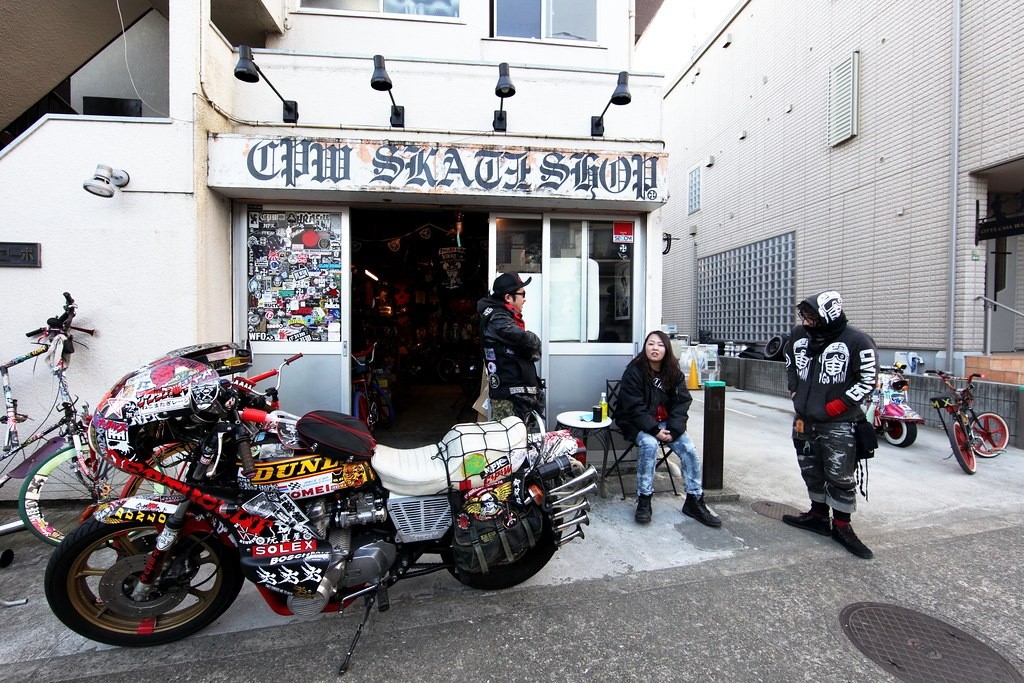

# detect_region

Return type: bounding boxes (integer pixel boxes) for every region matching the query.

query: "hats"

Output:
[493,271,532,296]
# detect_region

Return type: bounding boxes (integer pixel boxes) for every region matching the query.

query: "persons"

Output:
[615,330,723,527]
[782,290,880,558]
[477,272,541,431]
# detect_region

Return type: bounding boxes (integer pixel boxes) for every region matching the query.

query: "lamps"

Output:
[234,45,299,123]
[492,63,516,132]
[591,72,632,136]
[83,164,129,198]
[370,54,404,128]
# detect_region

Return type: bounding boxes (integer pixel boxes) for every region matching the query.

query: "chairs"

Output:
[599,379,681,501]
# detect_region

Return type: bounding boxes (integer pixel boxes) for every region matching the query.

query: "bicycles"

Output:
[925,369,1010,474]
[0,292,168,546]
[350,343,396,439]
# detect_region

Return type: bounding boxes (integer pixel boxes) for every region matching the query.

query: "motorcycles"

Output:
[41,343,599,676]
[861,363,924,448]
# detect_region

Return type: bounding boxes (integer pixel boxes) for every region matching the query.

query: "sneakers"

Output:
[783,512,831,535]
[832,519,873,558]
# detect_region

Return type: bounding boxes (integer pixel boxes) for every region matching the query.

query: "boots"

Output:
[634,489,653,522]
[683,492,720,526]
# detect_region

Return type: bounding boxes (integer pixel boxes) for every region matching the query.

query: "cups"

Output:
[592,405,602,422]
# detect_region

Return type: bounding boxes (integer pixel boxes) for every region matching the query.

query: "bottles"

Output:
[598,392,607,421]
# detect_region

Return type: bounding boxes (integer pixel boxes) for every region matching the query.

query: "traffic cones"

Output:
[686,360,702,390]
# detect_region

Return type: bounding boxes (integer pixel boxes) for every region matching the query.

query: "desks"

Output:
[552,411,612,498]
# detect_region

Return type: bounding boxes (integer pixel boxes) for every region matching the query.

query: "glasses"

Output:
[505,290,525,298]
[798,310,819,325]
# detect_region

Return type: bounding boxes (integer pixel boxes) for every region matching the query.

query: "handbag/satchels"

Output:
[296,410,376,463]
[513,388,545,423]
[853,419,878,459]
[450,497,542,573]
